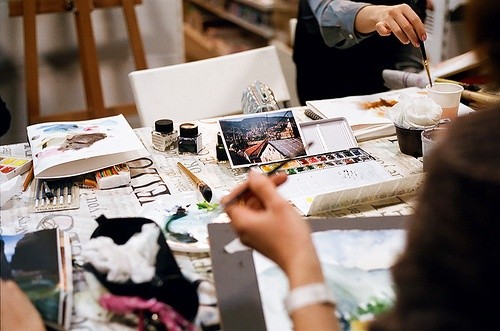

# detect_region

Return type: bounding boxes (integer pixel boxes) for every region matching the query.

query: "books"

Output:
[304,96,396,142]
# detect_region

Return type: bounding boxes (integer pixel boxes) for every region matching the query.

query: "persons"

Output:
[222,1,499,331]
[293,0,427,105]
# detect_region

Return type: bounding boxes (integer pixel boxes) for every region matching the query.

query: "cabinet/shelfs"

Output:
[183,0,298,64]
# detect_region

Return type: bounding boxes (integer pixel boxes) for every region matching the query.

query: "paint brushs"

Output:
[21,165,34,191]
[436,78,481,92]
[177,161,213,204]
[35,177,73,206]
[305,109,325,120]
[98,163,129,179]
[415,26,433,87]
[224,142,313,209]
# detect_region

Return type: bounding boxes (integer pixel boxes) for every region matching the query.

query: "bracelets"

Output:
[284,283,335,313]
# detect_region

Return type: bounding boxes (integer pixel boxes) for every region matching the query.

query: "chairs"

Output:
[128,46,290,128]
[9,0,147,125]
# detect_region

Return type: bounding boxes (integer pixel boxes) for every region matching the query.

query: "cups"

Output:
[426,82,464,120]
[394,120,439,158]
[420,128,445,172]
